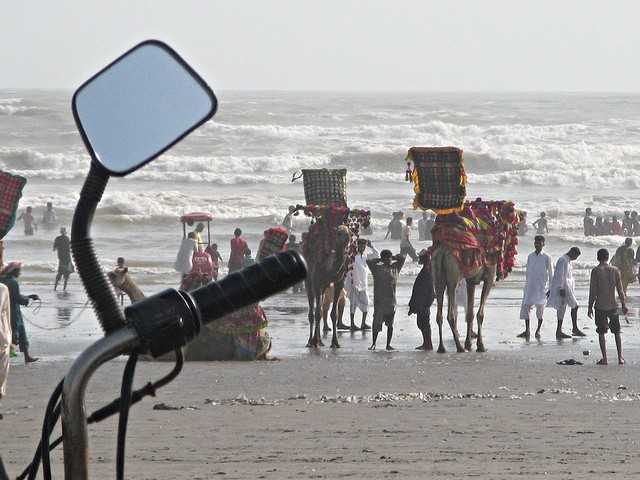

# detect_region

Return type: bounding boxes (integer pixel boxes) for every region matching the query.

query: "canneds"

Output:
[583,350,591,355]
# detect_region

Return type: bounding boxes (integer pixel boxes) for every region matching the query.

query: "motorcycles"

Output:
[0,37,308,480]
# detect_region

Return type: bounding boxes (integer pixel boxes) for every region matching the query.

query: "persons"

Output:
[52,226,75,291]
[0,283,13,420]
[194,222,205,252]
[583,207,640,236]
[281,205,296,235]
[227,228,249,275]
[407,248,436,350]
[587,249,628,364]
[365,250,406,351]
[616,238,634,297]
[384,212,403,239]
[516,235,555,339]
[17,206,38,235]
[455,278,477,338]
[348,238,380,331]
[212,243,223,272]
[173,232,198,274]
[532,211,548,234]
[517,214,528,236]
[114,256,126,306]
[255,230,307,293]
[544,246,586,338]
[322,280,350,331]
[240,249,254,269]
[0,261,40,364]
[418,212,429,241]
[400,217,418,262]
[42,201,57,224]
[424,214,436,240]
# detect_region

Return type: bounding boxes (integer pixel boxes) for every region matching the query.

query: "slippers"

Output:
[572,330,587,336]
[556,333,572,338]
[618,358,626,364]
[556,359,569,365]
[597,359,608,365]
[568,359,583,365]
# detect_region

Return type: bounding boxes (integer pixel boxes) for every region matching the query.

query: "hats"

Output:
[417,248,430,257]
